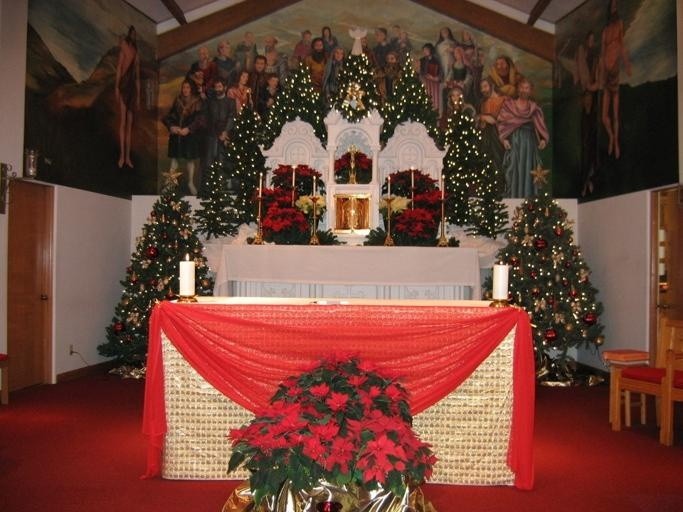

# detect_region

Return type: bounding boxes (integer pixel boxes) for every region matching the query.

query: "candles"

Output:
[177,252,196,298]
[491,262,509,301]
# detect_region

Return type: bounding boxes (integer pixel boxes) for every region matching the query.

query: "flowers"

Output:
[252,164,338,245]
[365,169,459,246]
[224,356,438,507]
[334,151,371,184]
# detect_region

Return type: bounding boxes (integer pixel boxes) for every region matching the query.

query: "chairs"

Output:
[610,314,682,446]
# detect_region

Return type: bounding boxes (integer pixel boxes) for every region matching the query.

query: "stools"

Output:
[603,349,649,428]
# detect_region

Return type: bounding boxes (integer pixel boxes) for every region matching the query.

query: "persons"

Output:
[573,42,604,196]
[114,25,141,171]
[599,1,634,160]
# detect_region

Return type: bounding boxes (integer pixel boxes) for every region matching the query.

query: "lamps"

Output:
[0,147,38,214]
